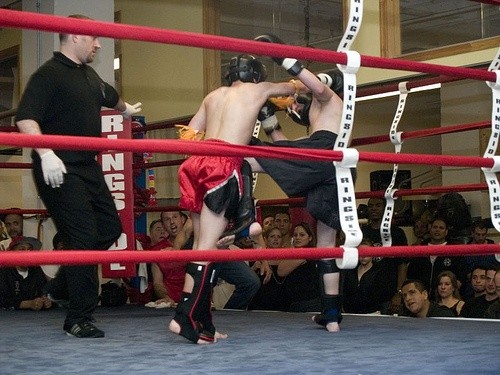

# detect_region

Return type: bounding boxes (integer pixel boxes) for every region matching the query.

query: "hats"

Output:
[7,236,42,251]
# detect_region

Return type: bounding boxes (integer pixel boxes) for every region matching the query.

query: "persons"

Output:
[169,55,344,345]
[15,15,142,337]
[217,34,356,333]
[0,194,500,320]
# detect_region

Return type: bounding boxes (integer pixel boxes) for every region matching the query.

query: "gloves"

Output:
[316,69,344,94]
[121,102,142,118]
[40,151,67,188]
[257,99,281,136]
[253,34,304,76]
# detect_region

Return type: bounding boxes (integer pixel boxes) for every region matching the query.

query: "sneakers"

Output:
[47,293,72,304]
[62,318,105,338]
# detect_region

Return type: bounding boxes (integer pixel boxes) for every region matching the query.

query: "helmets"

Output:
[284,93,314,127]
[225,54,267,86]
